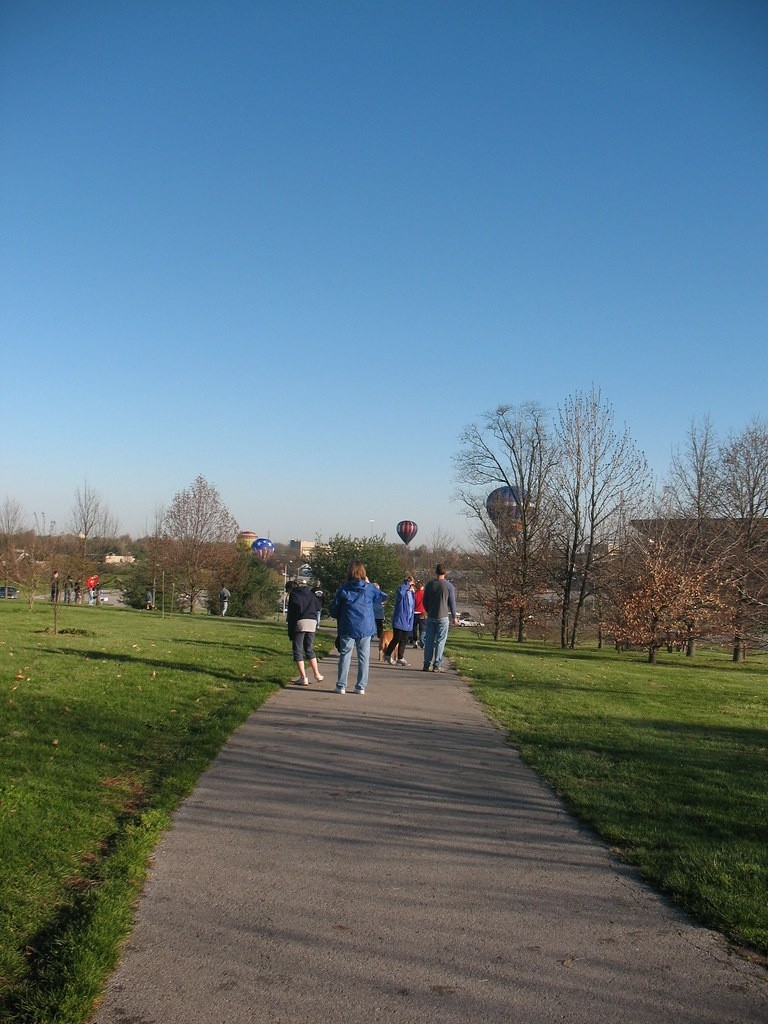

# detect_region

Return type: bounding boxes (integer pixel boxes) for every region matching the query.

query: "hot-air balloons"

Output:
[396,520,418,548]
[486,486,536,556]
[236,531,258,552]
[252,538,275,562]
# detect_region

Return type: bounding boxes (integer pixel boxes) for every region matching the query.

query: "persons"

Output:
[146,588,157,610]
[371,582,390,642]
[410,580,428,649]
[383,575,416,666]
[63,573,104,606]
[329,561,381,696]
[285,581,324,686]
[50,570,60,602]
[423,564,459,673]
[218,584,231,616]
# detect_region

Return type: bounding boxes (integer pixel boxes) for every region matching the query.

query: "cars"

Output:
[458,618,486,627]
[0,586,20,599]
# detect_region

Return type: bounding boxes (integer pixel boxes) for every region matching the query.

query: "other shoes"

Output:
[423,667,446,673]
[335,687,346,694]
[292,677,308,686]
[397,657,412,667]
[383,654,396,665]
[422,646,425,650]
[221,612,224,617]
[314,673,324,682]
[354,688,365,694]
[413,644,418,649]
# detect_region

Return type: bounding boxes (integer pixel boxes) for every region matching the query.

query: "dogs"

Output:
[377,629,397,662]
